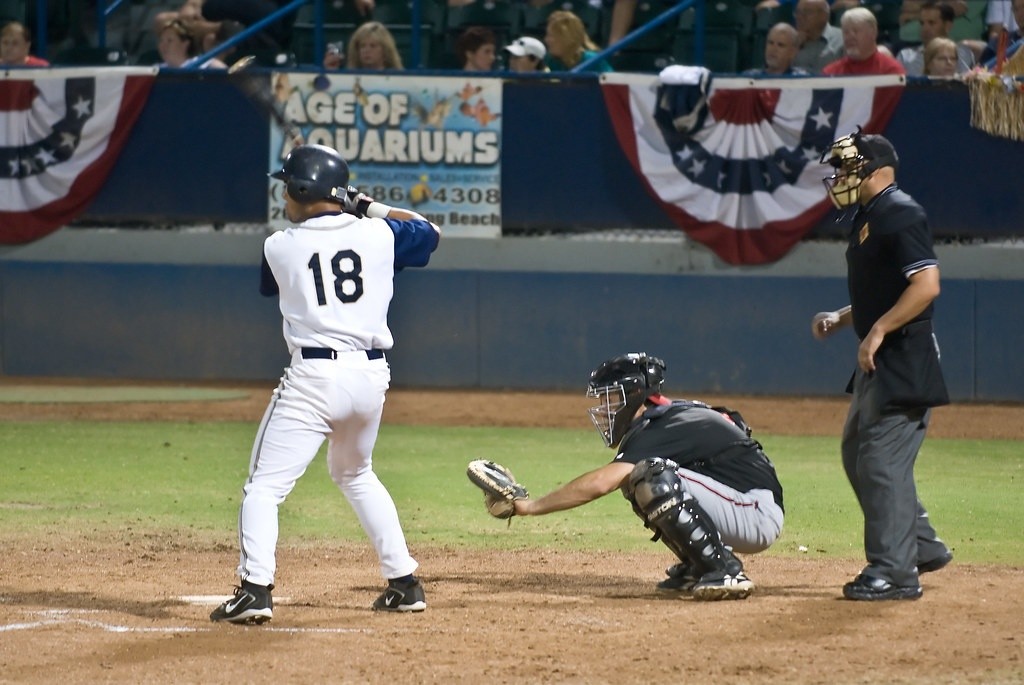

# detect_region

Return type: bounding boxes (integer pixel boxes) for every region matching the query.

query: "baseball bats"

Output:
[228,54,304,148]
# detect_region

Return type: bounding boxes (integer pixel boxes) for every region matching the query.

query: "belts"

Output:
[302,346,383,360]
[772,492,786,514]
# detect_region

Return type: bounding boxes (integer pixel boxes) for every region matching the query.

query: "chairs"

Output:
[320,24,356,70]
[674,29,739,77]
[383,25,432,71]
[758,7,796,31]
[749,31,769,70]
[715,3,766,41]
[449,30,509,72]
[292,23,318,72]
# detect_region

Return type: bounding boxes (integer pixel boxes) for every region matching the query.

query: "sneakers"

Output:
[372,578,426,611]
[917,550,953,576]
[209,582,276,625]
[692,569,754,602]
[654,561,700,591]
[842,573,924,601]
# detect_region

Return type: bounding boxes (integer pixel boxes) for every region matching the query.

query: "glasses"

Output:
[933,55,957,65]
[325,46,341,56]
[511,39,526,47]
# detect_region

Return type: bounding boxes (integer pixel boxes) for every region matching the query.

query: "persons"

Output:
[812,134,952,600]
[467,353,786,599]
[211,144,441,624]
[1,0,1024,244]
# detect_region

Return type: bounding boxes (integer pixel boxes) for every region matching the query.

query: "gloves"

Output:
[340,184,375,219]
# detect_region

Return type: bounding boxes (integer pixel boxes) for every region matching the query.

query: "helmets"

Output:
[587,351,668,448]
[266,144,350,203]
[819,124,900,181]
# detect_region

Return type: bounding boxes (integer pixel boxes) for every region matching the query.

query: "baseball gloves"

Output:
[467,459,529,521]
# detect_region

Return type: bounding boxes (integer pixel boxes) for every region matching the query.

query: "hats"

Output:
[501,36,547,60]
